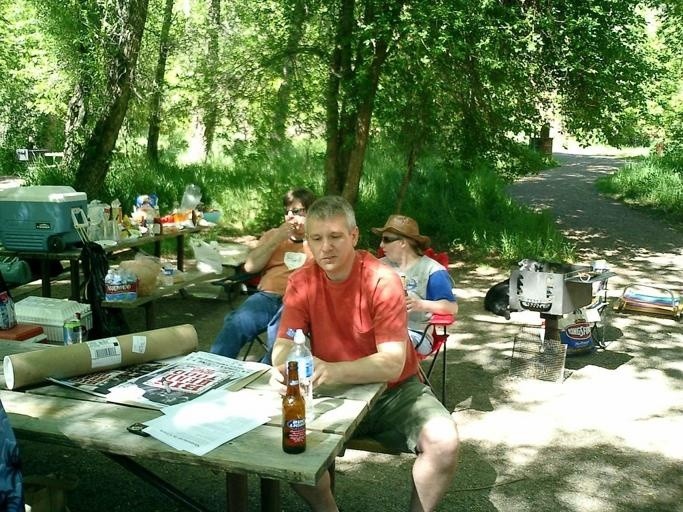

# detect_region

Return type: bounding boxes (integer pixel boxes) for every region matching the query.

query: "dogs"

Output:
[483,274,523,320]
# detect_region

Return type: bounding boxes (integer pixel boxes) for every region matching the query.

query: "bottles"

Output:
[145,211,154,237]
[283,330,315,424]
[281,360,307,453]
[73,312,87,345]
[163,259,173,287]
[105,269,135,299]
[400,276,408,310]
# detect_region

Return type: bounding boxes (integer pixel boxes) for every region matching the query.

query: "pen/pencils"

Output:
[161,377,171,394]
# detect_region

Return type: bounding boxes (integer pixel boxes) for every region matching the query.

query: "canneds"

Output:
[62,320,82,347]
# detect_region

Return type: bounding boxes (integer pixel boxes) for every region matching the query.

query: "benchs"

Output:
[326,434,417,495]
[99,266,234,330]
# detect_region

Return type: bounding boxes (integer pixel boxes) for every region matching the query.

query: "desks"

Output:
[0,336,388,512]
[0,226,203,302]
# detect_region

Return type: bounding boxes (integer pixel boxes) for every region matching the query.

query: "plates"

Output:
[94,239,117,248]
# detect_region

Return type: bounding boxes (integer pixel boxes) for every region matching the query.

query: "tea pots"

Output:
[102,219,121,242]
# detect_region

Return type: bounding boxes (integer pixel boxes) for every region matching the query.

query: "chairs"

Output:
[209,272,282,362]
[374,246,454,407]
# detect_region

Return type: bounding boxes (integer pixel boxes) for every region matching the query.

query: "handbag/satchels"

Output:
[1,256,34,284]
[1,291,17,330]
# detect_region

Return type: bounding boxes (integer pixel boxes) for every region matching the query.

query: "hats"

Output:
[371,214,430,245]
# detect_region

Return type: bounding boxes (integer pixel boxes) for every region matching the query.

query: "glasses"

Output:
[382,236,399,244]
[285,207,306,214]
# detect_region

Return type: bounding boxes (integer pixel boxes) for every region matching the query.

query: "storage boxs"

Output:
[507,259,617,316]
[0,184,87,252]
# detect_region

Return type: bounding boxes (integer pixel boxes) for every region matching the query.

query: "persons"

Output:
[371,215,458,356]
[271,196,459,512]
[209,188,317,366]
